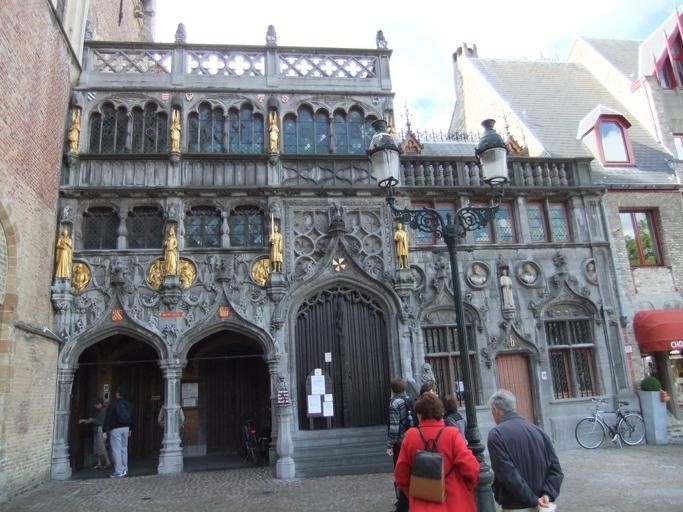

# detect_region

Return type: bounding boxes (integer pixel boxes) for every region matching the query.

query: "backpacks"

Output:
[400,396,419,428]
[408,426,457,503]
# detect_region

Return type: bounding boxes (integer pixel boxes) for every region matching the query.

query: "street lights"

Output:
[361,115,512,512]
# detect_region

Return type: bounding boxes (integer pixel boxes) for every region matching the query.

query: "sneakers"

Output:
[110,470,127,477]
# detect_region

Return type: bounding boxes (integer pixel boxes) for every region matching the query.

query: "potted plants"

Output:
[638,372,669,447]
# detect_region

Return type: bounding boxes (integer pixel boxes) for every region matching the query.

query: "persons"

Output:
[66,120,79,152]
[521,265,535,283]
[385,380,481,512]
[72,263,88,292]
[102,388,132,478]
[469,265,485,285]
[587,263,596,282]
[487,389,562,512]
[79,399,112,470]
[501,269,514,309]
[269,226,282,272]
[269,119,278,152]
[55,229,73,278]
[148,226,192,289]
[170,118,181,152]
[395,224,409,268]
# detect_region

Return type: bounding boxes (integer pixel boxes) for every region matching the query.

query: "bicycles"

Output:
[572,397,646,450]
[238,414,264,466]
